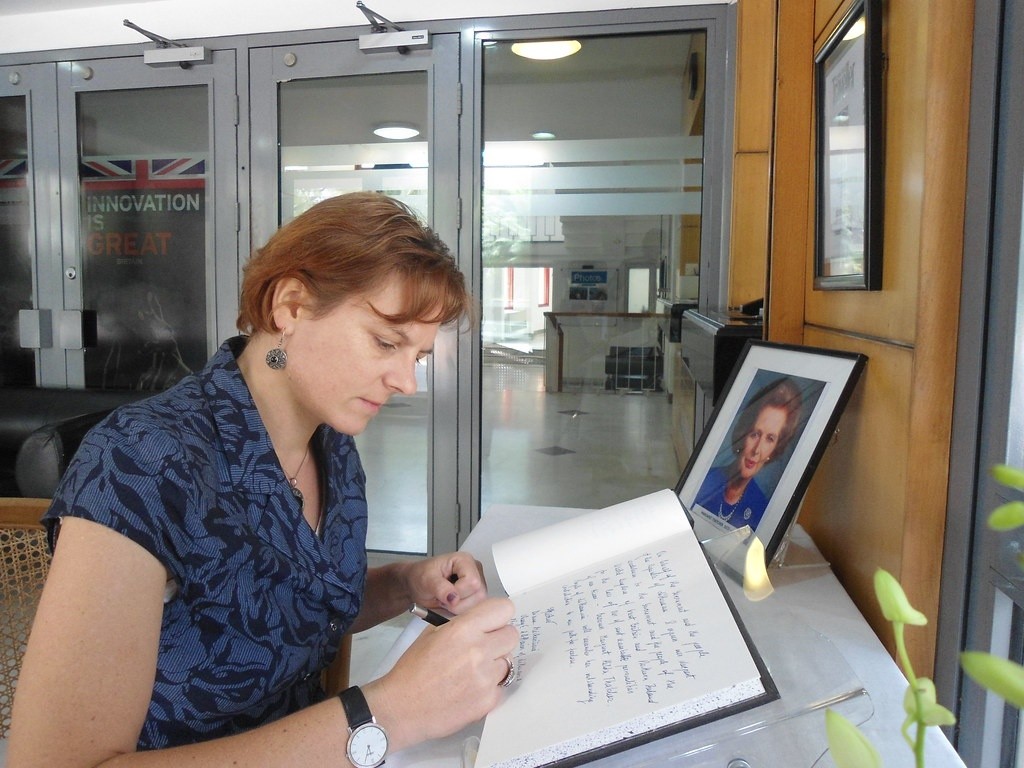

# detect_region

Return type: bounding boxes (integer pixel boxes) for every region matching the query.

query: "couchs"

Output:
[0,386,147,499]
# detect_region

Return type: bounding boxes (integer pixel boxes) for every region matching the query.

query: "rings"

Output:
[497,656,516,689]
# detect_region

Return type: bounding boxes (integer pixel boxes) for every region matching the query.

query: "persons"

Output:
[695,377,802,536]
[8,191,520,768]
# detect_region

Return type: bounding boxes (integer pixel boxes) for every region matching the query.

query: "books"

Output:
[473,488,780,768]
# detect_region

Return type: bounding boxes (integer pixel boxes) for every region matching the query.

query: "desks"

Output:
[364,501,971,767]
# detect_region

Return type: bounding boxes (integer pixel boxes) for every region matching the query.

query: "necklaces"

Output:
[282,443,311,514]
[718,482,742,522]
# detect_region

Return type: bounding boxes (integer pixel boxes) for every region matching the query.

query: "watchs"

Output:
[337,684,388,768]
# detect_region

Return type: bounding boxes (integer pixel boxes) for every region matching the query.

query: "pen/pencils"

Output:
[407,602,452,628]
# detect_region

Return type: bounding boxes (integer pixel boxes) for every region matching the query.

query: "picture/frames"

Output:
[674,337,868,590]
[809,1,883,293]
